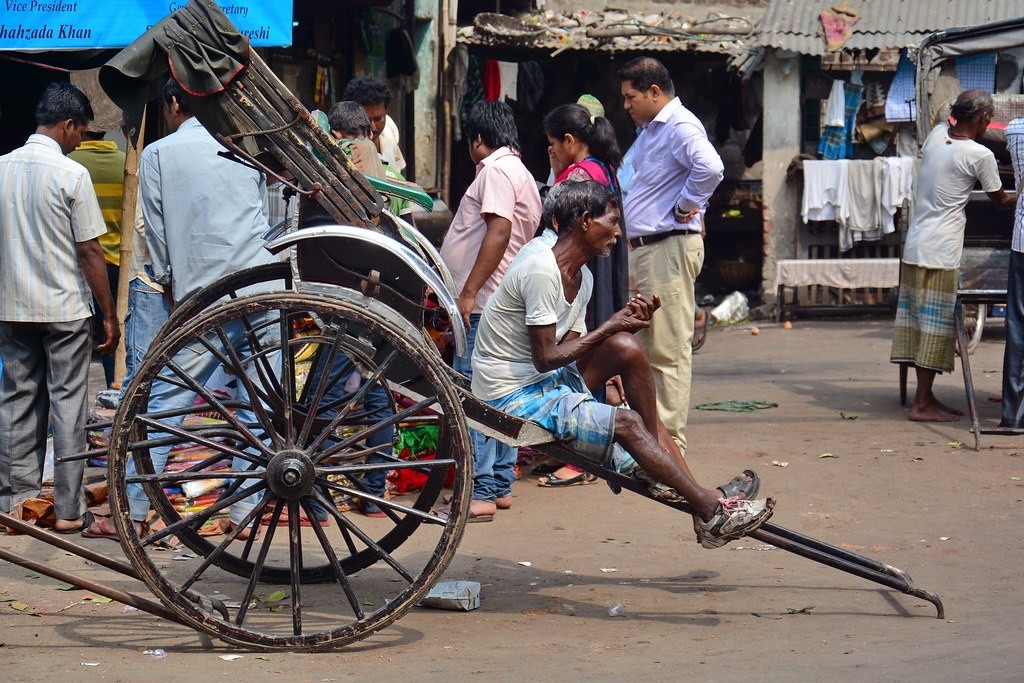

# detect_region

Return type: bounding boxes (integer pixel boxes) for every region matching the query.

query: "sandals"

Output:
[701,496,778,550]
[537,472,599,487]
[696,469,760,544]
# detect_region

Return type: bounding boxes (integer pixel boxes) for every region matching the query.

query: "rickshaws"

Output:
[900,14,1024,451]
[53,0,946,652]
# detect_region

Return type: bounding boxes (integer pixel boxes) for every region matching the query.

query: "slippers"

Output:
[422,504,494,524]
[357,503,388,519]
[443,494,511,510]
[81,520,120,540]
[56,510,103,535]
[260,507,329,527]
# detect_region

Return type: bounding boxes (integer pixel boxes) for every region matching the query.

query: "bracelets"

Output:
[676,203,690,214]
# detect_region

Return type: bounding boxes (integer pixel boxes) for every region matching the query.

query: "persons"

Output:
[471,182,775,549]
[66,131,124,387]
[263,77,408,527]
[889,89,1016,422]
[0,84,122,535]
[80,78,296,538]
[537,103,619,488]
[615,56,725,460]
[426,101,542,525]
[1001,118,1024,428]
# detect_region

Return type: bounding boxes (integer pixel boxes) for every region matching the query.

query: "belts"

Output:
[628,229,700,251]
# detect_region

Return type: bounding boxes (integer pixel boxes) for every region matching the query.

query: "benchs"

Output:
[932,94,1024,292]
[294,109,433,397]
